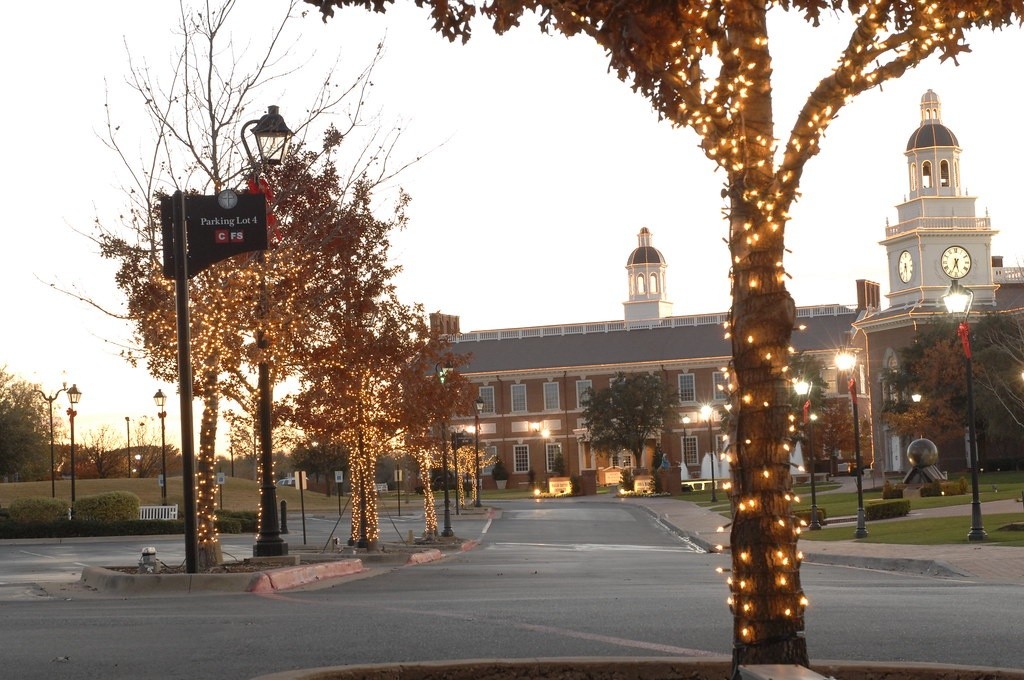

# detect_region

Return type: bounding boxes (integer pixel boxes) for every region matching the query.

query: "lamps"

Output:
[141,547,156,556]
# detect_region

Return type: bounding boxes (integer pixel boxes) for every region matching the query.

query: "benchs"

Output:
[138,504,178,520]
[376,483,388,492]
[681,481,718,491]
[792,473,827,484]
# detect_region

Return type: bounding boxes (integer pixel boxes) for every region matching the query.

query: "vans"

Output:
[275,477,309,486]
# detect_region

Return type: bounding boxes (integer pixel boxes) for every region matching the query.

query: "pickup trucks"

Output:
[415,476,473,491]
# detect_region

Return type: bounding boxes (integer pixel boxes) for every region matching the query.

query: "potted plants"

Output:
[492,461,509,490]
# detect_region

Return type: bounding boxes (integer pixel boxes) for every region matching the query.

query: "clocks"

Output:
[898,250,913,283]
[941,246,972,279]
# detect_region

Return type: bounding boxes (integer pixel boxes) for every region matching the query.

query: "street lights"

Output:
[34,382,68,498]
[834,345,870,538]
[66,384,82,520]
[153,389,167,506]
[683,413,690,469]
[471,397,485,507]
[700,404,718,502]
[240,106,295,557]
[794,370,822,531]
[941,280,989,542]
[434,360,454,536]
